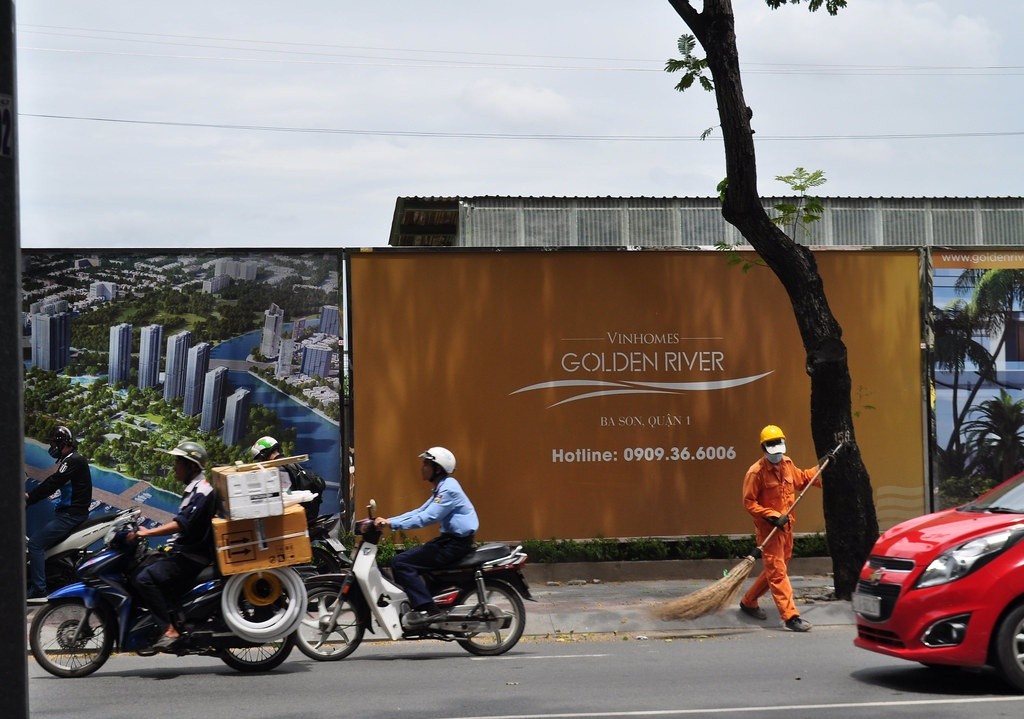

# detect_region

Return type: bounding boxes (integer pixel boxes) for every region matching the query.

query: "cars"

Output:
[849,465,1024,681]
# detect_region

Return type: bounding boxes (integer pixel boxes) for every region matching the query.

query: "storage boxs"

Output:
[211,464,284,521]
[211,503,313,576]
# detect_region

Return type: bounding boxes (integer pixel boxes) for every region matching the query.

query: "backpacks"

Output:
[283,460,326,522]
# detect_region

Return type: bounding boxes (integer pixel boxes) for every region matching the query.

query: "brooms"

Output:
[638,440,844,623]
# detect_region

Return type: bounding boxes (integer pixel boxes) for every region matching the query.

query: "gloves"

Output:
[818,449,838,469]
[771,515,789,530]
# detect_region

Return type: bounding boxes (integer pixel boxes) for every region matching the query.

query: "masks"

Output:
[765,452,782,463]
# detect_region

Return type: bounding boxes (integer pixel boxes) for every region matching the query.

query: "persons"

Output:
[373,446,480,626]
[251,436,325,528]
[739,424,841,632]
[125,442,217,652]
[24,425,93,603]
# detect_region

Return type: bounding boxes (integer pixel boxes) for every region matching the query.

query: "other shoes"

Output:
[26,590,49,602]
[788,614,812,631]
[407,608,447,624]
[152,635,182,649]
[740,600,768,619]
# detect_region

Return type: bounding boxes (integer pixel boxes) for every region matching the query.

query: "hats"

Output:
[763,439,787,455]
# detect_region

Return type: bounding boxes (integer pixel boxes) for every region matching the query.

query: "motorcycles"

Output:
[296,502,547,662]
[30,509,302,675]
[23,505,146,611]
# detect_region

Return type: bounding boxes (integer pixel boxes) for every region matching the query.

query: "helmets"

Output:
[251,436,279,460]
[419,446,456,474]
[45,425,73,446]
[168,442,207,470]
[759,425,787,446]
